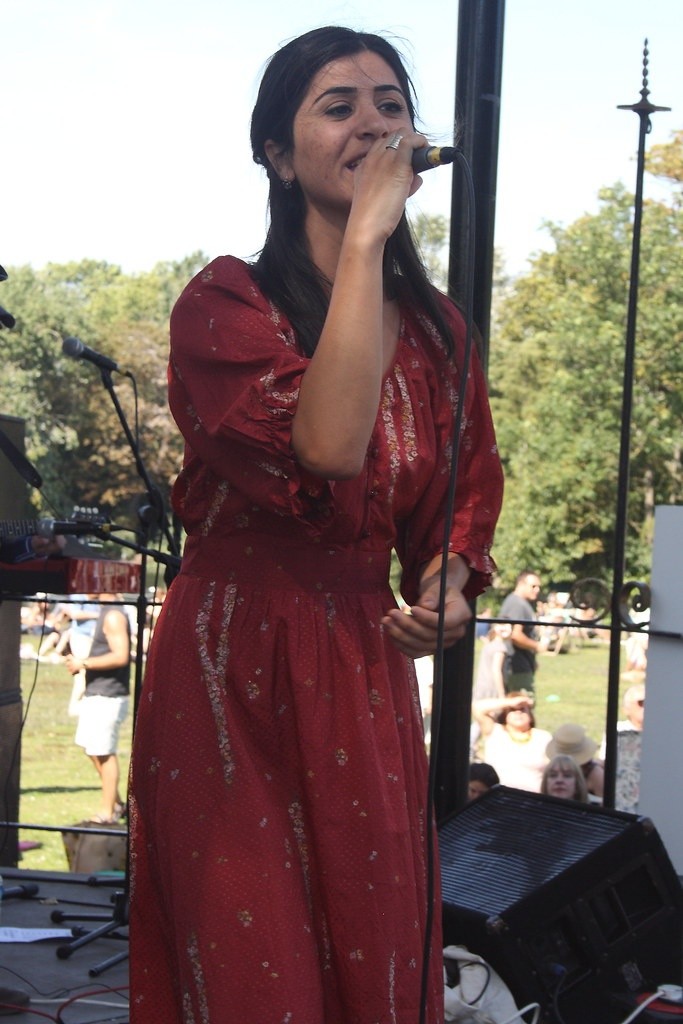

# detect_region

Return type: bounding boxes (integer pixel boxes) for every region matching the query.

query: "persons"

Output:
[20,590,165,823]
[468,568,652,802]
[131,27,503,1024]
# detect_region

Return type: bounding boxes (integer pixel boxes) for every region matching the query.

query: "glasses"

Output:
[634,699,646,709]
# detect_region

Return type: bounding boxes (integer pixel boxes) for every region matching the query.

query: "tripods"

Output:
[50,367,181,960]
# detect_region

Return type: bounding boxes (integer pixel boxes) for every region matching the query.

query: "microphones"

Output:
[411,144,460,175]
[37,517,123,539]
[61,337,133,378]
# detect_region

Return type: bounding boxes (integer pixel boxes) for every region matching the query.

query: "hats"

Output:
[545,722,601,766]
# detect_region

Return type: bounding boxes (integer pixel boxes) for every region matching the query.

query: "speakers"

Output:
[438,784,683,1024]
